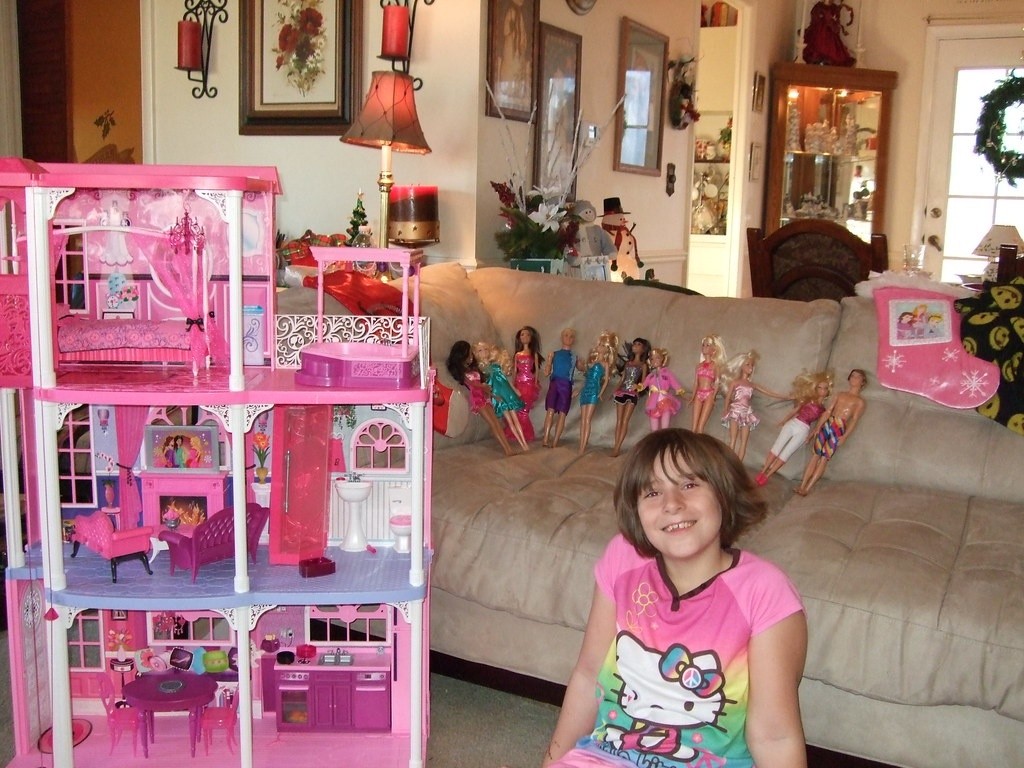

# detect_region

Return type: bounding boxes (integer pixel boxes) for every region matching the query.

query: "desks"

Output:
[138,471,228,546]
[109,658,134,709]
[121,672,215,759]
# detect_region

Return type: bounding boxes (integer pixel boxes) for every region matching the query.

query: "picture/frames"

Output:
[239,0,363,136]
[144,425,220,474]
[111,610,128,622]
[748,72,766,183]
[486,0,670,206]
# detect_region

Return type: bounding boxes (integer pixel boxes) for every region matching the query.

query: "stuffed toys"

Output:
[565,200,617,281]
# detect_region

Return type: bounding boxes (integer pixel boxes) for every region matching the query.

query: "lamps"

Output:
[153,611,186,634]
[972,225,1024,281]
[177,0,439,281]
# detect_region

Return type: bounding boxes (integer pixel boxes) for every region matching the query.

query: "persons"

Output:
[445,324,868,496]
[541,428,809,768]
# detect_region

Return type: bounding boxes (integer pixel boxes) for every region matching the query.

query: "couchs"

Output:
[277,264,1024,768]
[72,503,270,582]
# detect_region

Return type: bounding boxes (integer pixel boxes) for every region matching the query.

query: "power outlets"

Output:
[287,630,295,640]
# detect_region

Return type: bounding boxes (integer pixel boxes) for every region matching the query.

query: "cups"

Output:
[903,244,925,273]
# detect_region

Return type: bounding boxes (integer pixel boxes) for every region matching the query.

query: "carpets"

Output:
[38,718,92,754]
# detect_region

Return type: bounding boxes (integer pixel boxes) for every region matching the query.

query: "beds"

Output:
[55,305,197,367]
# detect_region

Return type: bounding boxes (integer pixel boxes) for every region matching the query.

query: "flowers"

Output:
[106,627,134,648]
[251,432,271,468]
[482,87,628,257]
[162,507,178,520]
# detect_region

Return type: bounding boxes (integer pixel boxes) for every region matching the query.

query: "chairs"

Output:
[747,216,889,304]
[95,673,240,756]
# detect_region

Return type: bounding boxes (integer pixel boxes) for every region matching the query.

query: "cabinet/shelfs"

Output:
[275,670,392,734]
[770,59,897,246]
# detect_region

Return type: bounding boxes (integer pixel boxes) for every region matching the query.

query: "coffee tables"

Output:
[149,524,197,563]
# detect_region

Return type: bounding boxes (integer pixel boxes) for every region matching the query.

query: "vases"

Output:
[117,645,125,661]
[164,519,180,532]
[513,255,565,277]
[256,467,268,484]
